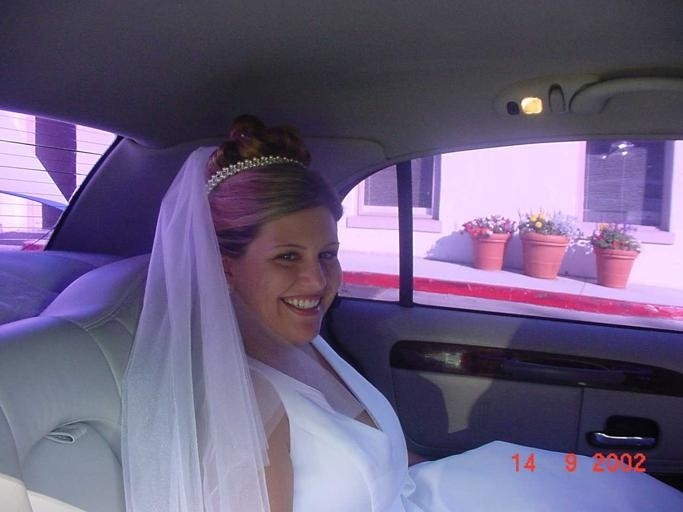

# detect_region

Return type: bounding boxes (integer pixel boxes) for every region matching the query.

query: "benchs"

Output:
[0,251,156,509]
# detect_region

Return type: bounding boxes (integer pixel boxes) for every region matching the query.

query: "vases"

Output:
[471,233,639,289]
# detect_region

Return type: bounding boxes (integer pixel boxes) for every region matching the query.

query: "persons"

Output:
[118,111,679,512]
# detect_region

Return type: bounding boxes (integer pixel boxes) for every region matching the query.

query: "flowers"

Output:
[462,207,642,254]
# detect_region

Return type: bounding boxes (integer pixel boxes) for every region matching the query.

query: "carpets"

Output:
[340,272,682,322]
